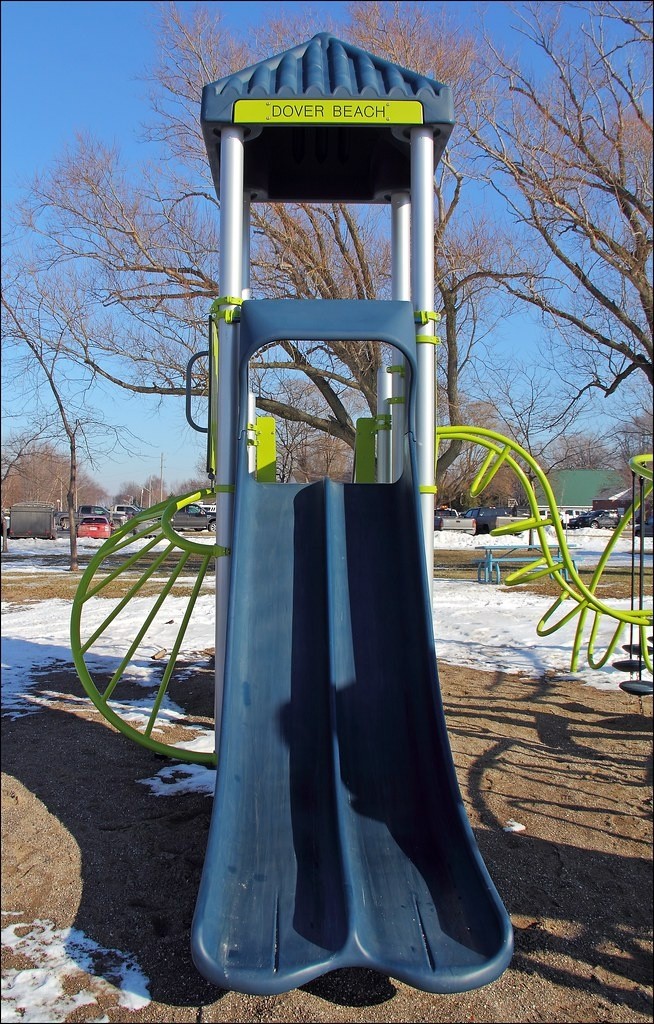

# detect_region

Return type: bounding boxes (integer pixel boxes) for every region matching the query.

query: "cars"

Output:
[432,502,654,537]
[0,494,218,541]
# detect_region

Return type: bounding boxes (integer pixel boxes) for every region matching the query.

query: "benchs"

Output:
[470,543,579,586]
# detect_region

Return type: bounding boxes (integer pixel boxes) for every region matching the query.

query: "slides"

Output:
[191,432,515,995]
